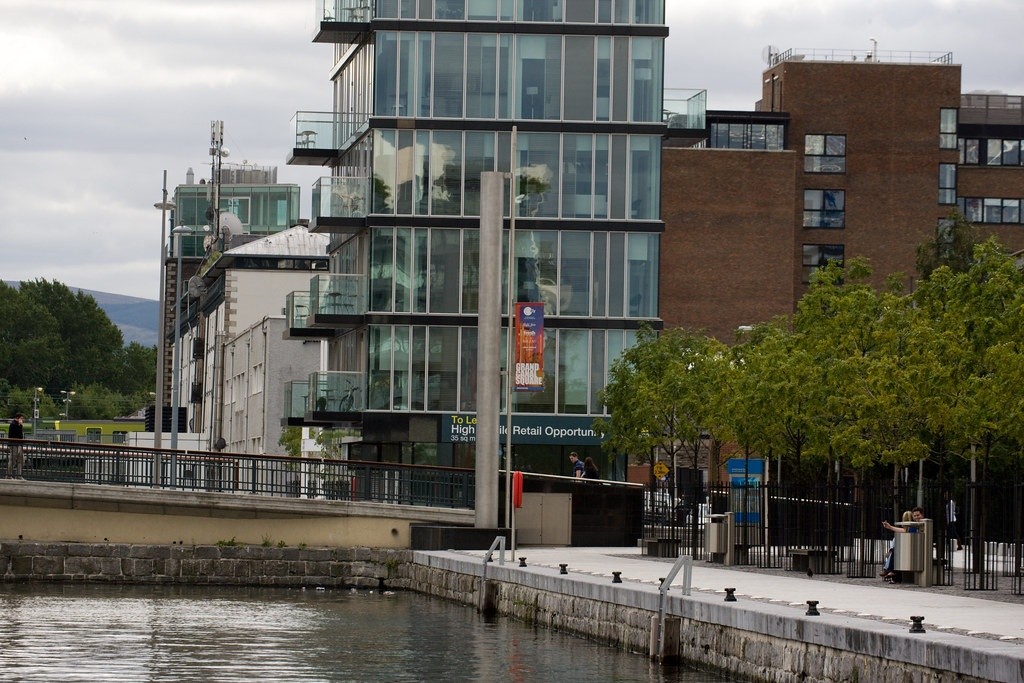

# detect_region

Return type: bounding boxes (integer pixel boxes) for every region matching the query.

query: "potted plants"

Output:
[314,428,350,501]
[277,427,316,498]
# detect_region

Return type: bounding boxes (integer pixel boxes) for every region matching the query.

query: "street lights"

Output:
[32,387,43,440]
[60,390,76,420]
[153,169,175,490]
[169,219,193,490]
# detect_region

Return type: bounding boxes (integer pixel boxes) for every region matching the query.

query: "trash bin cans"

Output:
[894,518,937,588]
[703,510,735,567]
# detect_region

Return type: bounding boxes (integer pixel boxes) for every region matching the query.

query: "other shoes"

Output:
[879,569,889,576]
[886,573,897,578]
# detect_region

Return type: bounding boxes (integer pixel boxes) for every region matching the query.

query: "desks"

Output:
[342,7,358,22]
[329,292,341,314]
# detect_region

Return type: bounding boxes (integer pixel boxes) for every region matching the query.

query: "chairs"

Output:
[301,130,318,149]
[342,294,357,314]
[358,6,370,22]
[324,8,336,22]
[319,294,328,314]
[294,305,310,328]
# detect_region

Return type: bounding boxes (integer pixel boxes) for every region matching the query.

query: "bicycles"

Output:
[339,378,361,413]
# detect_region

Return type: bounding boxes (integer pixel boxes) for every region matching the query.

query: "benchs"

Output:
[712,544,752,564]
[645,536,682,558]
[788,548,838,573]
[884,552,948,585]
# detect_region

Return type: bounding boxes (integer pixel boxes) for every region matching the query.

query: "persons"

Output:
[569,452,584,481]
[878,507,923,578]
[581,457,600,485]
[5,413,25,480]
